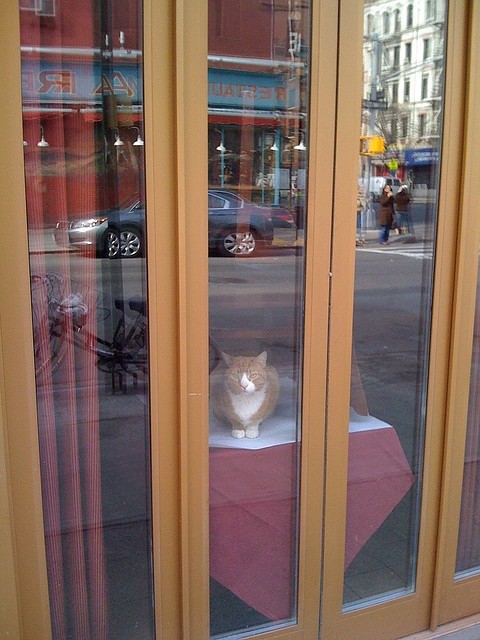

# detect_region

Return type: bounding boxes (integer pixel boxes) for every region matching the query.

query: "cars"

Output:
[54,189,296,257]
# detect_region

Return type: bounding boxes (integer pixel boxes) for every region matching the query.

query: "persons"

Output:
[396,181,416,237]
[374,174,396,250]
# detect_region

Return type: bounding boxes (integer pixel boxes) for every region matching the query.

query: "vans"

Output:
[372,177,405,197]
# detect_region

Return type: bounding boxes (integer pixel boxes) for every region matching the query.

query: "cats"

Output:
[208,351,280,439]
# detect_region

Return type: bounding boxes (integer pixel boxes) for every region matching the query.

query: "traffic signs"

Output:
[359,35,387,238]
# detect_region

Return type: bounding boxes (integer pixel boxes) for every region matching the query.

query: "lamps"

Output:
[214,128,228,151]
[113,127,125,146]
[291,131,307,151]
[131,126,144,146]
[37,123,50,146]
[266,133,279,150]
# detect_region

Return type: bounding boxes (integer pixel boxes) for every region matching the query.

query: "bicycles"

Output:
[27,271,221,393]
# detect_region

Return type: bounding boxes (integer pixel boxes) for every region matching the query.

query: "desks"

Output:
[138,374,415,621]
